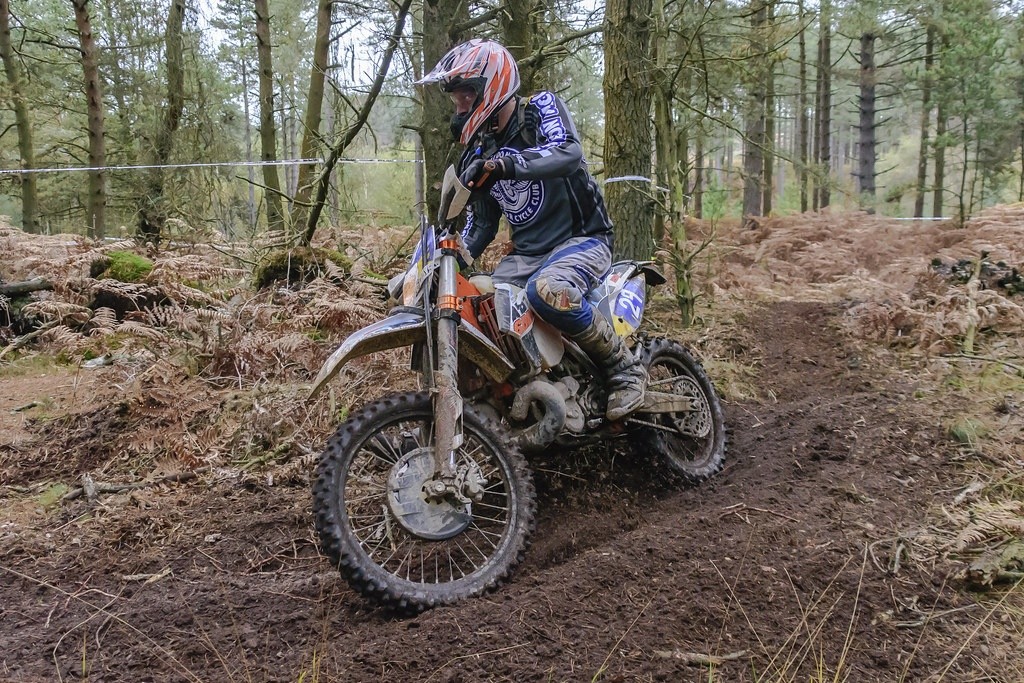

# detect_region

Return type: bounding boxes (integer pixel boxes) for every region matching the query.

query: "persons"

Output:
[422,41,649,418]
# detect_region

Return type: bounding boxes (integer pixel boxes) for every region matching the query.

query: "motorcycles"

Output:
[304,141,727,613]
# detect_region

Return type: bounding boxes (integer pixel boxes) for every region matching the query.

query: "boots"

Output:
[568,302,651,421]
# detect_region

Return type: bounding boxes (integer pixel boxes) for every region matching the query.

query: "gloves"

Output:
[458,155,515,206]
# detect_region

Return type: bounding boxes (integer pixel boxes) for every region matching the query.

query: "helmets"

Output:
[413,39,520,146]
